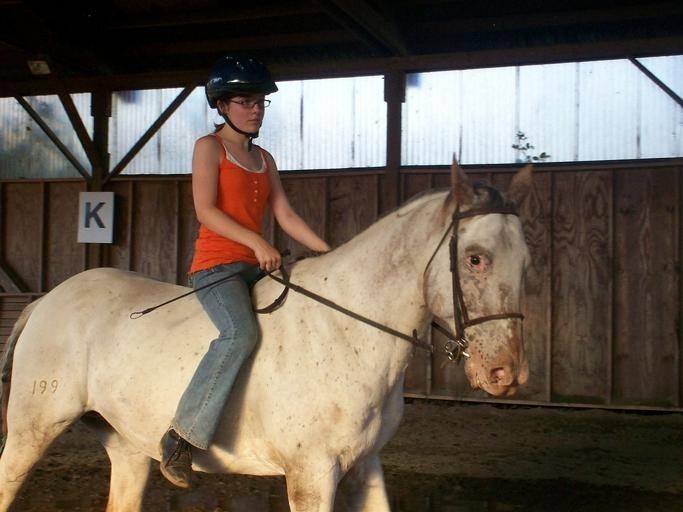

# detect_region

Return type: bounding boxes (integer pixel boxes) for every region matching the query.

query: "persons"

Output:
[155,50,330,493]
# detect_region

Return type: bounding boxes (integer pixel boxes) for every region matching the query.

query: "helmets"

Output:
[205,56,278,108]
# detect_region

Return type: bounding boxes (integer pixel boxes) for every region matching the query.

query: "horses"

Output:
[1,153,533,512]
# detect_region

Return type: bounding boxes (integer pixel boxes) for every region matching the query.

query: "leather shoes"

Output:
[159,425,198,488]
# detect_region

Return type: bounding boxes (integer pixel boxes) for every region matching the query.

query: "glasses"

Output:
[231,100,271,109]
